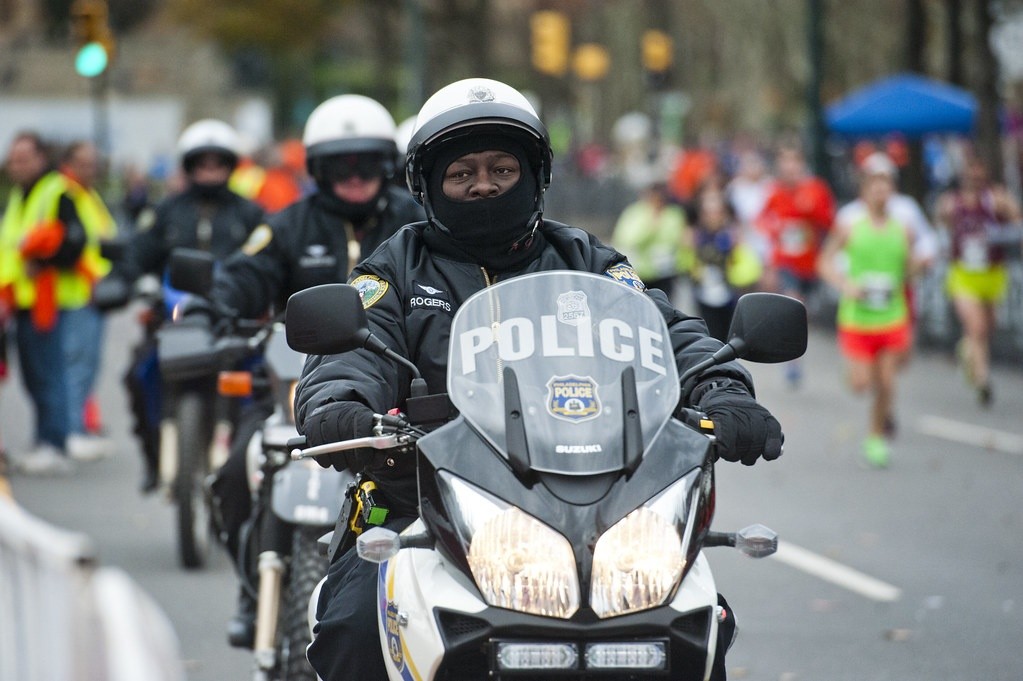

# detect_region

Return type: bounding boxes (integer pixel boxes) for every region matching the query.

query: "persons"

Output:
[215,92,429,649]
[289,78,783,681]
[0,131,120,474]
[101,114,274,493]
[595,147,836,400]
[812,151,943,467]
[931,152,1023,409]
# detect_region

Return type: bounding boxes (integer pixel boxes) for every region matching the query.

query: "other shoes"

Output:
[68,433,107,461]
[20,442,71,475]
[228,607,256,647]
[979,381,995,410]
[865,436,890,469]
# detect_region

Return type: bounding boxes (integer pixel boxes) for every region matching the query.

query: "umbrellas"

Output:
[824,69,990,136]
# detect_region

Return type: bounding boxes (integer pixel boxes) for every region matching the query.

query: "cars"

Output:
[915,224,1023,360]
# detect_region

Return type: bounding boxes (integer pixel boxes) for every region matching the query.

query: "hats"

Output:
[861,151,899,180]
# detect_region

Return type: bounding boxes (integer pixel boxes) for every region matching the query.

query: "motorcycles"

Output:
[94,237,808,681]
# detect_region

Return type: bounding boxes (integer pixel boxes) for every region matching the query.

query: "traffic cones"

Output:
[83,397,102,434]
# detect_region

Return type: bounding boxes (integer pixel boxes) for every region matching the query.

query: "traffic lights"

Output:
[68,0,115,76]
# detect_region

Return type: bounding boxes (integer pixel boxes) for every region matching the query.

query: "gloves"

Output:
[156,310,213,361]
[696,378,783,467]
[304,401,375,475]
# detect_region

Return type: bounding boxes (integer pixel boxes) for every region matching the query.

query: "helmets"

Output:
[404,78,553,203]
[178,119,248,172]
[301,90,400,171]
